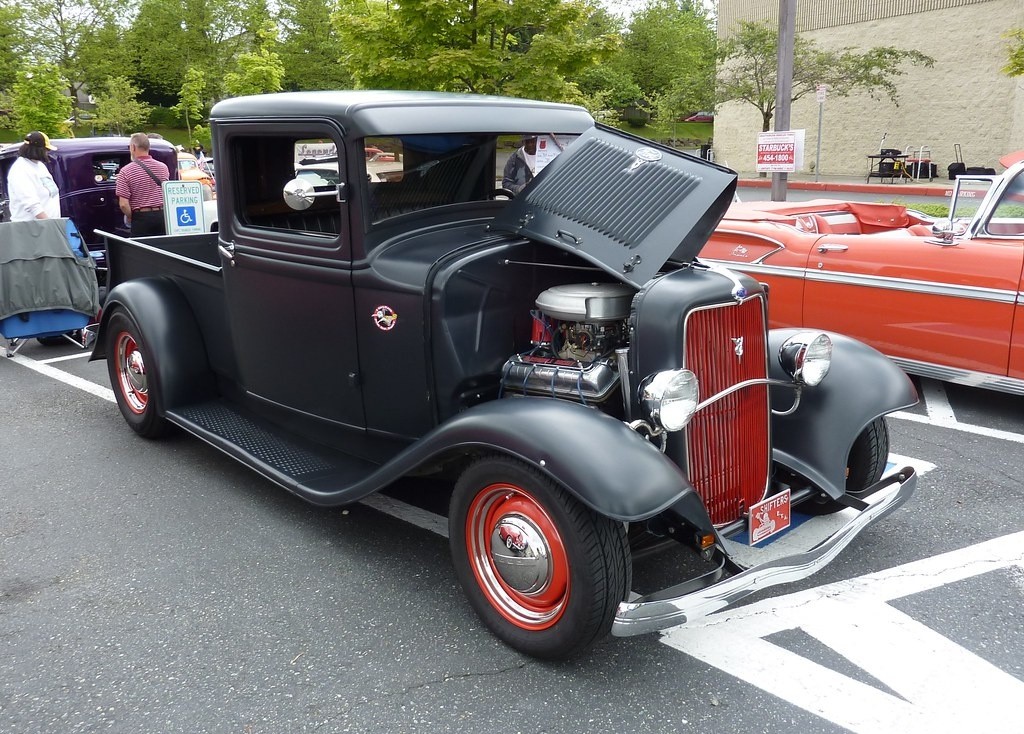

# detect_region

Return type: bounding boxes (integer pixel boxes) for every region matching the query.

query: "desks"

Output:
[866,155,911,184]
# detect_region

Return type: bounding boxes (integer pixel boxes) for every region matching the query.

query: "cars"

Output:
[87,89,922,665]
[1,133,179,346]
[695,150,1024,405]
[176,143,404,201]
[683,110,713,124]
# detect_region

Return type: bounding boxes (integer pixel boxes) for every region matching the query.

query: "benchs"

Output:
[247,202,452,234]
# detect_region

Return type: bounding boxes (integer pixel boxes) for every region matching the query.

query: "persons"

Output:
[502,135,537,195]
[147,133,184,153]
[7,130,63,222]
[192,139,203,159]
[115,133,170,238]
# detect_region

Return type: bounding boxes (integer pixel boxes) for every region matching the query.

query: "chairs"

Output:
[899,144,932,180]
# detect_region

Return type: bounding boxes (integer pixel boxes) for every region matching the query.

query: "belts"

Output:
[134,207,163,212]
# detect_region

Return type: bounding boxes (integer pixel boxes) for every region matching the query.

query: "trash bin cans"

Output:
[701,144,712,160]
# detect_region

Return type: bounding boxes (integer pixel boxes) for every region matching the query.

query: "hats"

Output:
[523,134,538,141]
[24,130,57,151]
